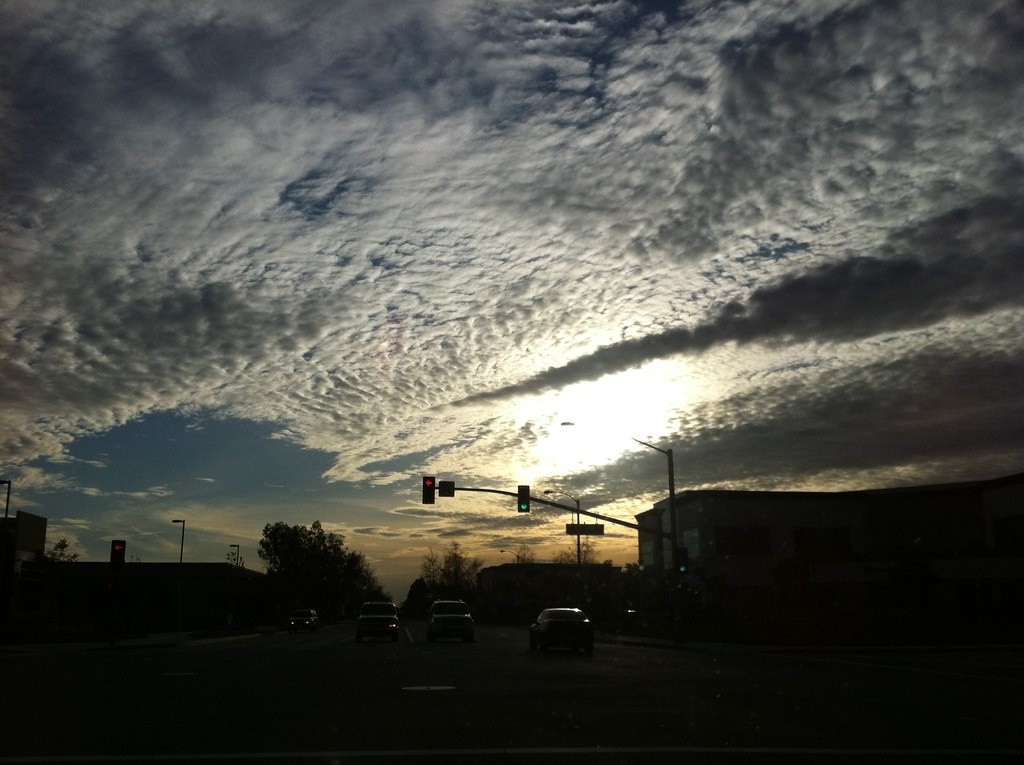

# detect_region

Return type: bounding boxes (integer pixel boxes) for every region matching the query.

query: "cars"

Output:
[528,608,594,651]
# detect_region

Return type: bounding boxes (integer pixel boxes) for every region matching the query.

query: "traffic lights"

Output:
[677,547,688,576]
[423,476,435,504]
[106,579,117,595]
[110,540,126,567]
[518,486,530,512]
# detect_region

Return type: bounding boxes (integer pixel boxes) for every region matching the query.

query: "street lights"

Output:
[0,480,12,575]
[172,519,185,642]
[230,544,240,565]
[560,420,687,645]
[544,490,581,565]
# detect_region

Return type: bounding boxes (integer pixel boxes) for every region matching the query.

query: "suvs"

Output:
[355,601,400,642]
[288,609,319,634]
[427,598,475,642]
